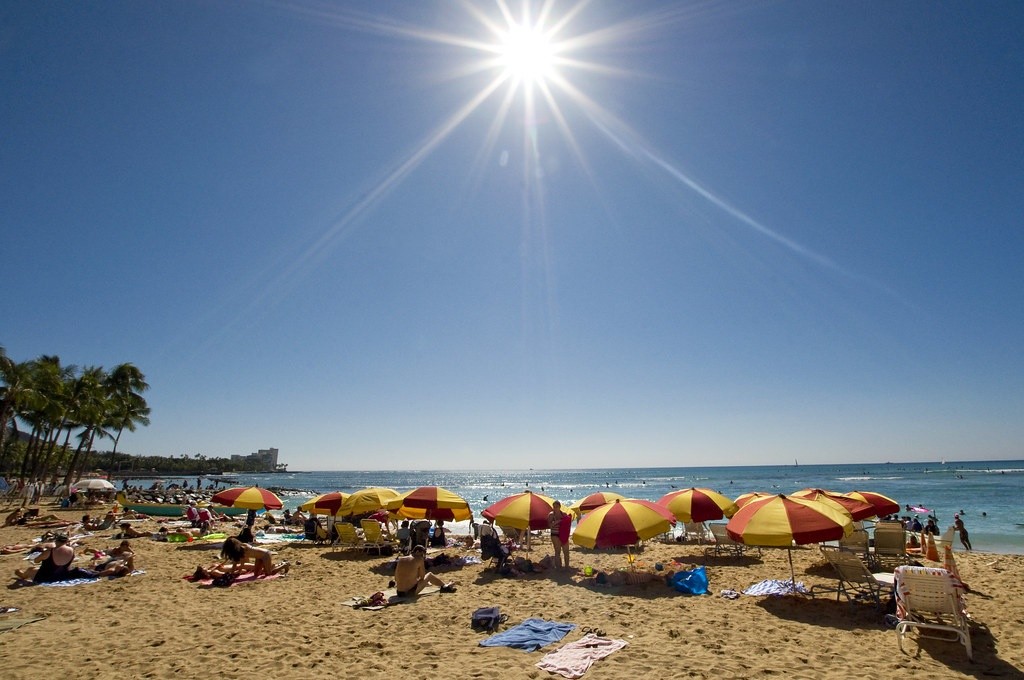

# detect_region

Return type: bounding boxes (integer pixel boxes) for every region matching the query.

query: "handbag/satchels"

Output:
[471,606,499,632]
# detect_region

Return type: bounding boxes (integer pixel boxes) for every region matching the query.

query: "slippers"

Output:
[592,627,607,636]
[578,627,591,637]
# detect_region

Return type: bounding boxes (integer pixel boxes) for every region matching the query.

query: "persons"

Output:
[0,473,291,590]
[905,501,924,512]
[885,513,973,549]
[861,467,1006,480]
[958,509,966,515]
[982,511,986,516]
[283,469,737,605]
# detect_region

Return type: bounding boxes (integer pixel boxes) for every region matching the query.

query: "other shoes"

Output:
[440,586,457,593]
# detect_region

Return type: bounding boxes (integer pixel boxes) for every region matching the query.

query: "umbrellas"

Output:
[386,485,470,560]
[658,480,739,545]
[573,496,675,570]
[727,486,854,601]
[481,491,574,560]
[336,480,403,521]
[71,479,114,489]
[568,486,622,510]
[798,478,901,521]
[298,491,348,521]
[210,481,284,528]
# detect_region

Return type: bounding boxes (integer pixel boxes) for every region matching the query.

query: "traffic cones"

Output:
[919,530,928,555]
[926,531,941,564]
[943,543,969,592]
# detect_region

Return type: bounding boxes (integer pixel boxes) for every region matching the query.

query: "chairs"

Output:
[305,514,528,568]
[838,523,870,568]
[895,565,975,658]
[872,519,911,566]
[820,543,896,609]
[705,523,738,558]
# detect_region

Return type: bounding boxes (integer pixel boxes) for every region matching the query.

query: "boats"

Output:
[115,492,248,517]
